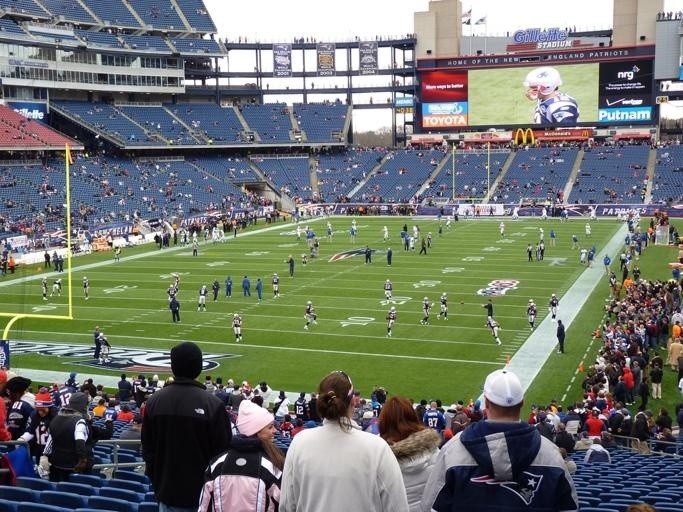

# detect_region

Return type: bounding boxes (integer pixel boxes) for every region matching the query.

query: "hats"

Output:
[52,371,285,405]
[170,342,203,380]
[68,393,88,411]
[483,369,524,407]
[35,391,54,409]
[0,369,8,384]
[5,376,31,393]
[235,399,274,437]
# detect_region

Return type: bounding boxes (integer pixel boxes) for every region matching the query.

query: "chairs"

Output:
[2,100,354,234]
[2,399,163,509]
[565,440,682,508]
[270,416,319,455]
[2,2,225,59]
[353,144,680,207]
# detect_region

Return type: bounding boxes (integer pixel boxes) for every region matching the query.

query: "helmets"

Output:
[523,66,564,101]
